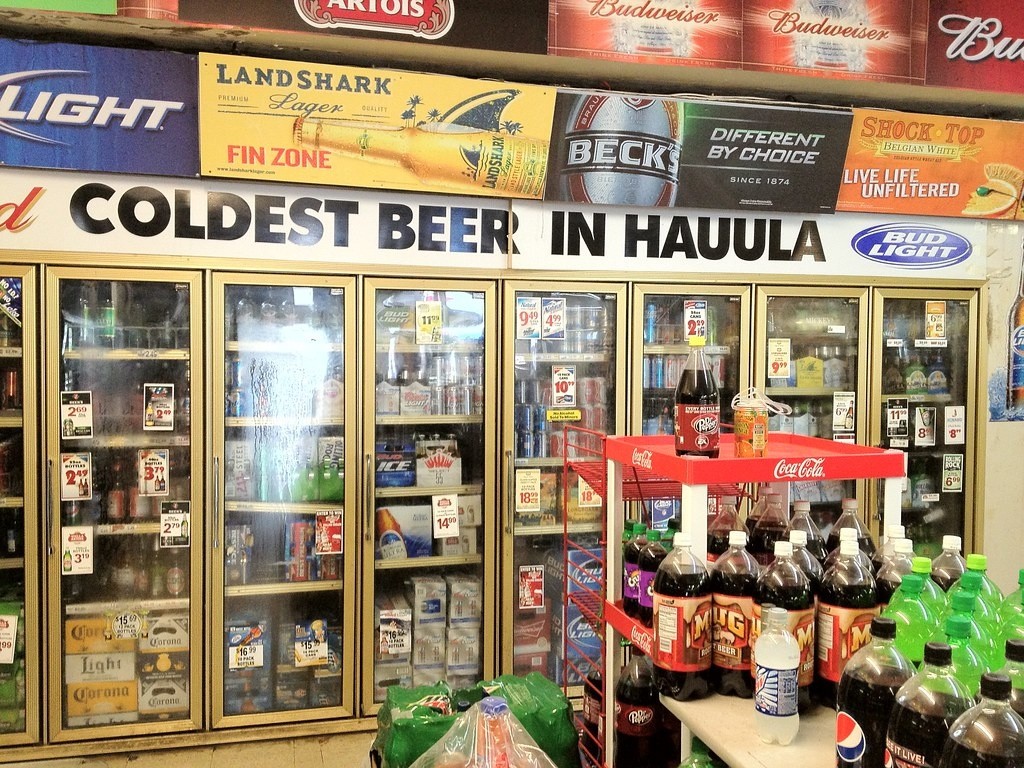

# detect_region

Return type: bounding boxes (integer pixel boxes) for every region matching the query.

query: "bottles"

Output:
[919,408,935,442]
[675,335,720,459]
[845,401,853,429]
[768,341,854,388]
[66,361,190,435]
[766,398,833,440]
[882,349,950,396]
[236,623,267,654]
[64,535,189,598]
[375,432,460,487]
[225,290,344,343]
[60,280,189,348]
[292,114,551,198]
[181,510,188,541]
[583,487,1024,768]
[64,452,190,524]
[6,506,21,558]
[374,671,581,768]
[376,508,407,559]
[1006,237,1024,409]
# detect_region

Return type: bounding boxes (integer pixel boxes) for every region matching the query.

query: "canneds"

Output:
[431,301,724,459]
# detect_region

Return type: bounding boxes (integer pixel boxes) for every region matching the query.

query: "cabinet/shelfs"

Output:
[563,423,906,768]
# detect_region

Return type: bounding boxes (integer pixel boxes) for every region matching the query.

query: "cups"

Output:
[311,621,328,642]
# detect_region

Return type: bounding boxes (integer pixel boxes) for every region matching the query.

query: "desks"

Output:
[660,692,838,768]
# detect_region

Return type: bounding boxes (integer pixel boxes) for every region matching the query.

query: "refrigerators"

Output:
[0,263,983,746]
